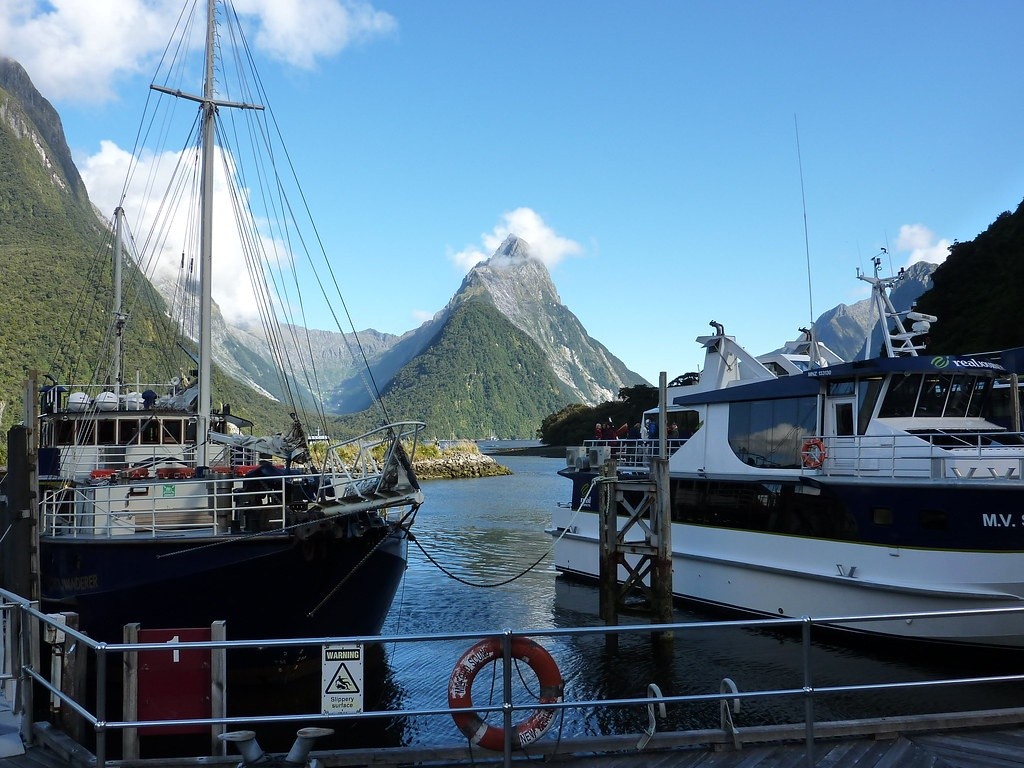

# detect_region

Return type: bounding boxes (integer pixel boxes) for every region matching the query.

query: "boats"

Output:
[542,109,1023,654]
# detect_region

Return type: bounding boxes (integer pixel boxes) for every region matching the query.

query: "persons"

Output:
[595,414,658,467]
[667,421,679,452]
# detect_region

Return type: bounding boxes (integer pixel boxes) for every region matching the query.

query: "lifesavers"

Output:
[446,632,565,754]
[801,439,826,468]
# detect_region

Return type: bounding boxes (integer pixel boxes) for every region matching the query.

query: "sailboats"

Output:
[0,0,427,695]
